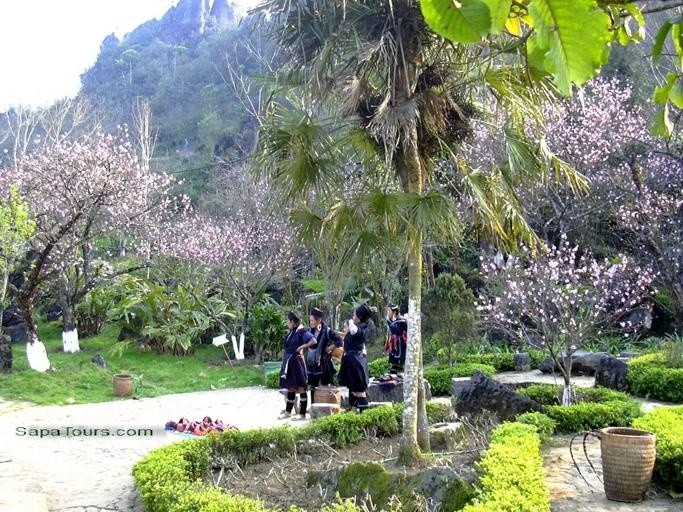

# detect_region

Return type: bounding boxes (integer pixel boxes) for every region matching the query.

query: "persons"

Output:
[343,316,367,356]
[336,303,377,412]
[279,309,318,420]
[385,303,401,374]
[306,307,341,404]
[388,302,408,375]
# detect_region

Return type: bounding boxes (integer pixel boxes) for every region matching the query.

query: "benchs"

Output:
[620,352,642,360]
[341,377,431,408]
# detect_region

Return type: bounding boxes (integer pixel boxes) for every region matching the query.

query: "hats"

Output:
[354,303,374,324]
[308,307,324,318]
[286,310,301,324]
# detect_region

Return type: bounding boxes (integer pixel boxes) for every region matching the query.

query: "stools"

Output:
[309,403,339,423]
[616,358,629,364]
[370,401,394,411]
[513,353,531,371]
[452,377,472,397]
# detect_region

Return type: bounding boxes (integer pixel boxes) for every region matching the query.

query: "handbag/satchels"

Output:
[305,344,323,368]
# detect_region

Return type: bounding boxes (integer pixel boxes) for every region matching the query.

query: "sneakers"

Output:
[290,412,305,422]
[275,409,292,421]
